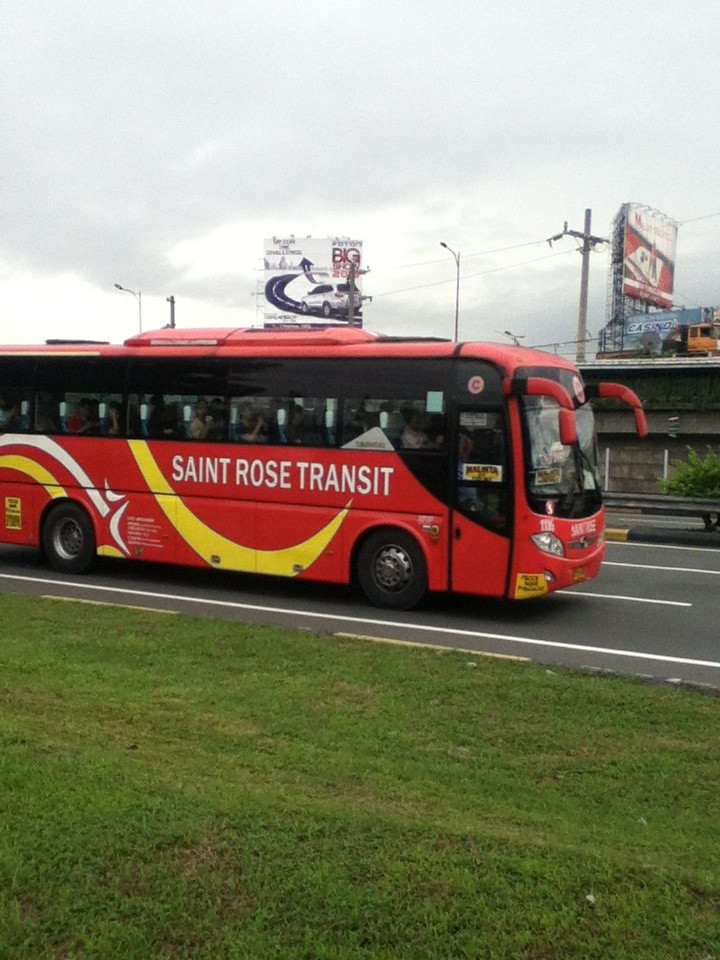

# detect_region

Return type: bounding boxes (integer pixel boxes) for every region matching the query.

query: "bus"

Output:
[2,326,649,614]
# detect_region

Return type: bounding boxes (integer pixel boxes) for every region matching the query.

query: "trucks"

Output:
[687,322,720,356]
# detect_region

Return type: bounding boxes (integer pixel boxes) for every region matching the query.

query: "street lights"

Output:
[115,284,142,334]
[441,242,460,343]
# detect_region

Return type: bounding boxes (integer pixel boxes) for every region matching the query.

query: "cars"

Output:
[301,282,363,317]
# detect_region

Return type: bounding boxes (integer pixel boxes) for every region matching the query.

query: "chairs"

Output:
[23,401,404,445]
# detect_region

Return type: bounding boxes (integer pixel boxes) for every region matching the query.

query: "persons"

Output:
[1,391,482,509]
